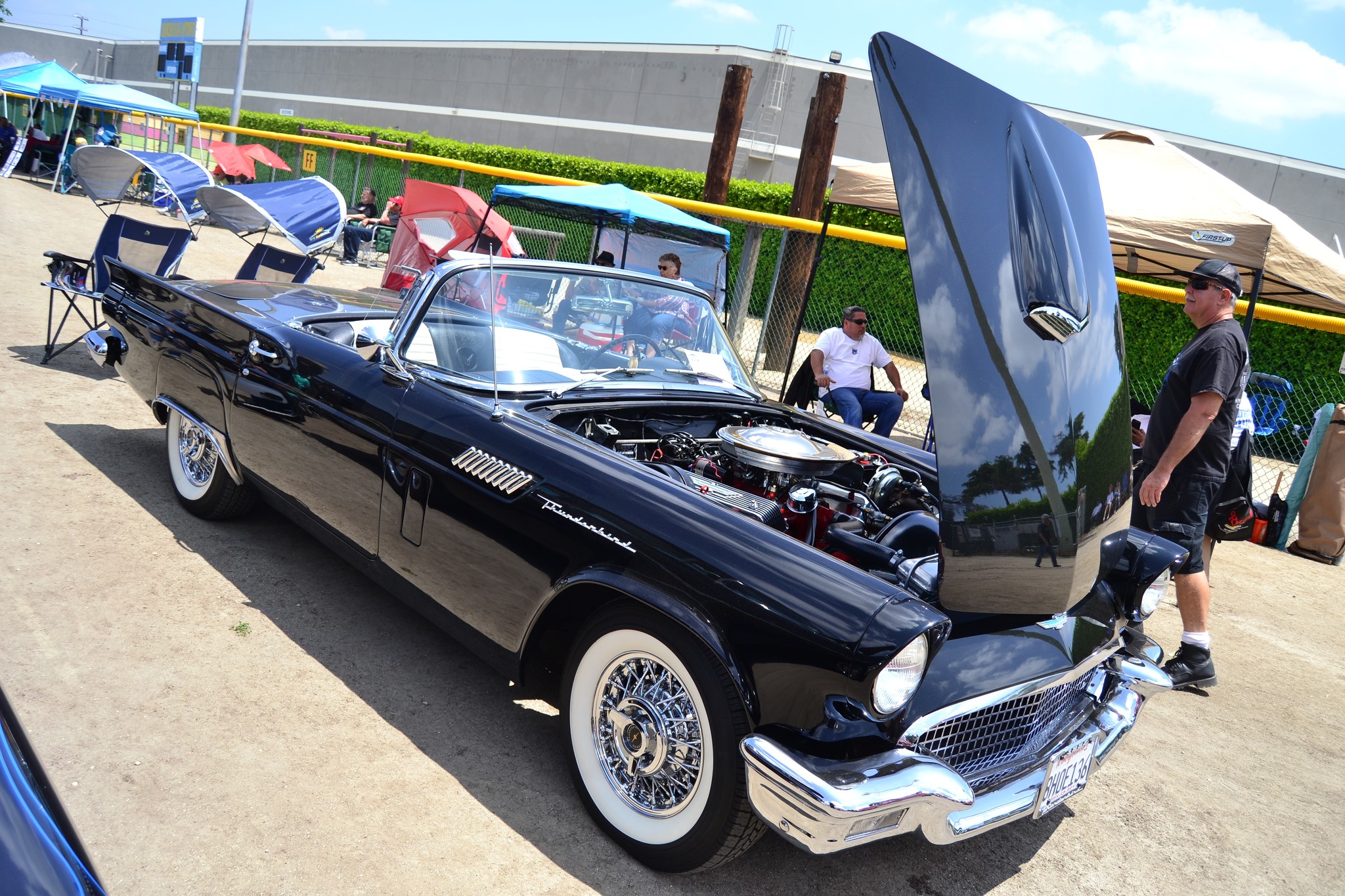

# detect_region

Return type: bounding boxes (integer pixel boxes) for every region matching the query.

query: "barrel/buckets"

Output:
[1247,515,1268,543]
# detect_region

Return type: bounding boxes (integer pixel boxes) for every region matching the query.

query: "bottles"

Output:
[145,196,153,201]
[823,363,831,376]
[507,302,539,317]
[95,127,104,139]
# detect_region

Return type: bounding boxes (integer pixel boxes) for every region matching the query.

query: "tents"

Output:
[0,51,43,72]
[490,182,731,252]
[38,83,200,120]
[833,124,1345,314]
[0,60,89,100]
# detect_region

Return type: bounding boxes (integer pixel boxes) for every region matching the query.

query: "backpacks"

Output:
[1204,429,1257,543]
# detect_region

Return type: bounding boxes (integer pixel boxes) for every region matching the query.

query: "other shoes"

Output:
[341,259,359,267]
[336,256,350,262]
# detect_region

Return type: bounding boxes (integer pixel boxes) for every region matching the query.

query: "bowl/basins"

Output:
[637,297,644,300]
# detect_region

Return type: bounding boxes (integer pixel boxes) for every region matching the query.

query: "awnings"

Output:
[197,175,348,255]
[71,144,215,237]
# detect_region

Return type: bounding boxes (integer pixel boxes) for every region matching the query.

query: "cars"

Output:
[84,29,1193,875]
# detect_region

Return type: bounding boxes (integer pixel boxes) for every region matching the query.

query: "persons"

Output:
[1114,482,1121,514]
[552,250,616,335]
[0,115,87,151]
[287,370,310,398]
[1090,500,1102,531]
[1035,514,1062,568]
[60,136,89,192]
[623,253,692,363]
[336,195,405,266]
[1103,487,1113,522]
[810,306,909,438]
[1131,260,1253,690]
[1131,412,1153,449]
[156,164,254,215]
[309,187,377,255]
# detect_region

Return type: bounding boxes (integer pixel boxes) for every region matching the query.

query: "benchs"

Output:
[326,319,582,374]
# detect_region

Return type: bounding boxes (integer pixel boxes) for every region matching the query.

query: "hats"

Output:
[388,196,404,205]
[595,250,616,267]
[74,127,86,136]
[1174,259,1242,298]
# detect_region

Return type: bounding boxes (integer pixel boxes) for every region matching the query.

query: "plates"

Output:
[626,295,644,302]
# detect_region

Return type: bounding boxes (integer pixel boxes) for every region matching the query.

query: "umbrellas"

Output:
[207,141,257,180]
[381,178,528,316]
[240,142,293,172]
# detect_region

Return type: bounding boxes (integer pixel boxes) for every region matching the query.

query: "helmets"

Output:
[75,137,87,146]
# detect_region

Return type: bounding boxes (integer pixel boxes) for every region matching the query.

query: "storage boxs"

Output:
[577,322,623,353]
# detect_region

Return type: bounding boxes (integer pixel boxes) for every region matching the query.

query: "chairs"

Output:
[170,243,320,288]
[495,254,562,330]
[661,301,699,365]
[427,253,491,305]
[39,215,192,365]
[139,172,171,208]
[335,217,396,270]
[811,363,878,429]
[34,148,59,177]
[921,381,936,454]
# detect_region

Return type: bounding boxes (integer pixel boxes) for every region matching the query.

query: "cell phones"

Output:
[1131,419,1141,435]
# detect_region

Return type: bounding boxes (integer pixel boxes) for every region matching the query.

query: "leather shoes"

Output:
[1160,641,1218,690]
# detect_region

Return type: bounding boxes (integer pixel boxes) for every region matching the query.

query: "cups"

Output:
[10,137,15,144]
[1292,424,1301,435]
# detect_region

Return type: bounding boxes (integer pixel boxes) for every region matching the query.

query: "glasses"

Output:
[846,319,868,325]
[1187,276,1234,299]
[658,265,673,271]
